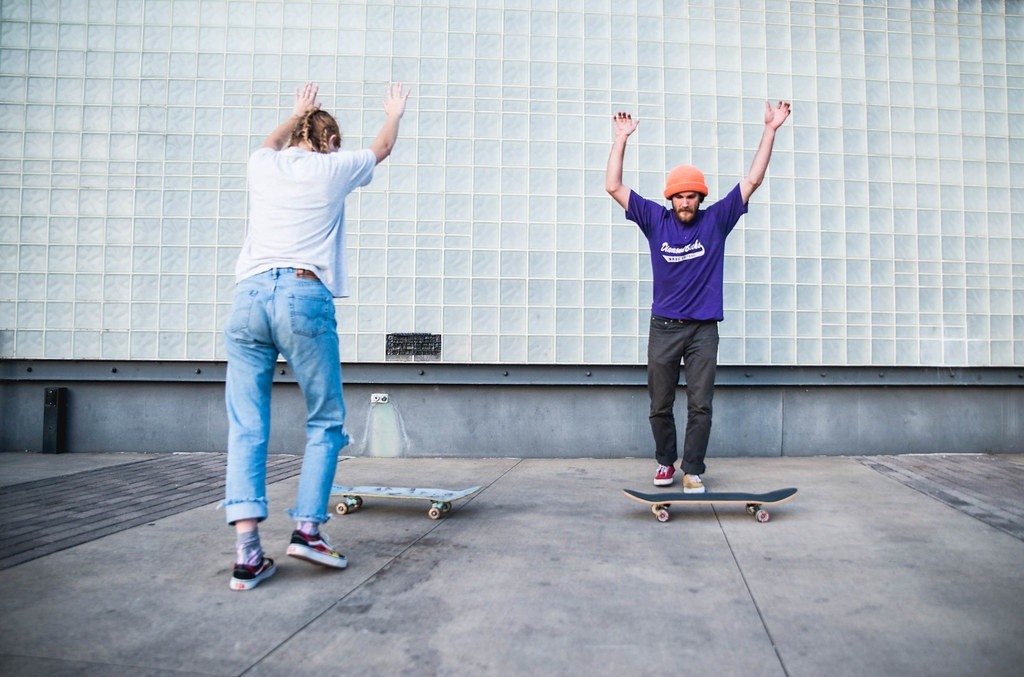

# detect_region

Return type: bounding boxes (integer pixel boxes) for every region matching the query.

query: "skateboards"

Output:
[621,486,799,523]
[326,484,485,520]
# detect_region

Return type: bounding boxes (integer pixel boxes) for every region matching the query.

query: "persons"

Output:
[216,80,413,591]
[603,102,793,493]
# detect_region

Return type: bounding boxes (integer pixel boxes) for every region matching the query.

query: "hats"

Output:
[663,164,708,199]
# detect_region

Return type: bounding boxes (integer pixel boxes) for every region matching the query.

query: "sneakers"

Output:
[653,464,676,485]
[230,551,277,590]
[286,529,347,568]
[683,474,706,494]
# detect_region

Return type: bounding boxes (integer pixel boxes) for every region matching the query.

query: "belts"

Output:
[653,316,698,324]
